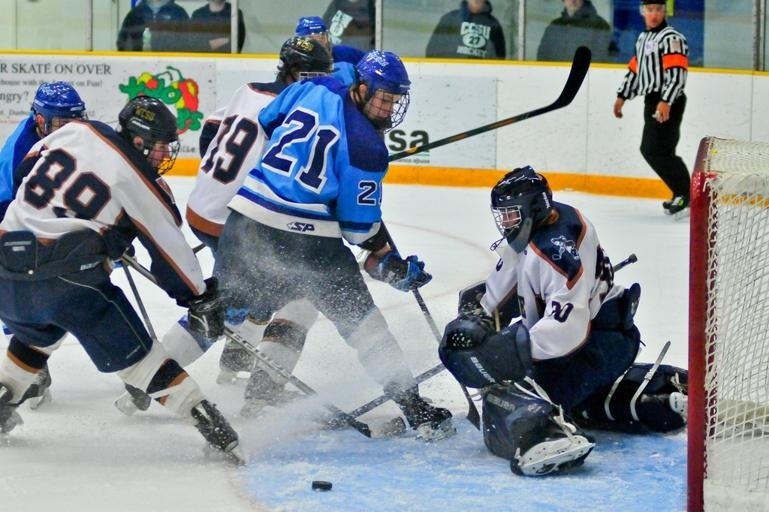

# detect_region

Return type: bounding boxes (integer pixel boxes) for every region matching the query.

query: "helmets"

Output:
[30,81,86,140]
[118,94,181,181]
[488,165,556,255]
[295,15,333,56]
[279,35,335,84]
[351,49,411,134]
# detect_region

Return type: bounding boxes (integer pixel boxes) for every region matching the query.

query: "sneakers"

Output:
[218,336,260,375]
[26,364,52,399]
[124,382,154,412]
[508,414,596,478]
[662,191,690,215]
[190,397,240,454]
[383,383,454,432]
[641,392,688,432]
[0,382,21,434]
[243,365,303,409]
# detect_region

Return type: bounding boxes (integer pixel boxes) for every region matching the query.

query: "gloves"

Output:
[363,251,433,293]
[176,275,225,339]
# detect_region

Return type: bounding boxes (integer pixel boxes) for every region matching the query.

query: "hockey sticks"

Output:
[123,252,371,438]
[318,361,446,426]
[381,215,481,429]
[387,47,591,162]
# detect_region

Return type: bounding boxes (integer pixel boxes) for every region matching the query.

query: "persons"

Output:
[440,165,690,474]
[425,0,505,59]
[127,52,453,430]
[0,93,242,456]
[190,1,246,54]
[0,82,90,400]
[324,0,376,53]
[116,0,189,52]
[186,33,331,414]
[537,0,617,62]
[614,1,691,214]
